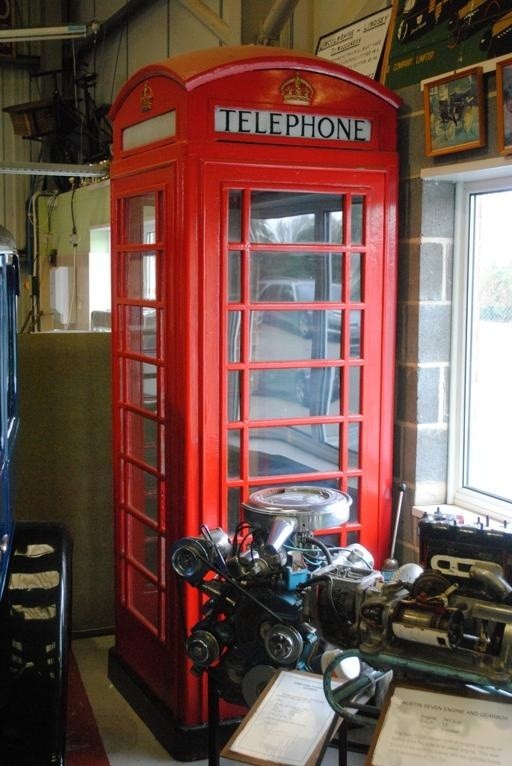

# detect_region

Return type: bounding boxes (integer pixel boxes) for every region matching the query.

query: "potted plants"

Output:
[422,65,486,157]
[495,56,512,155]
